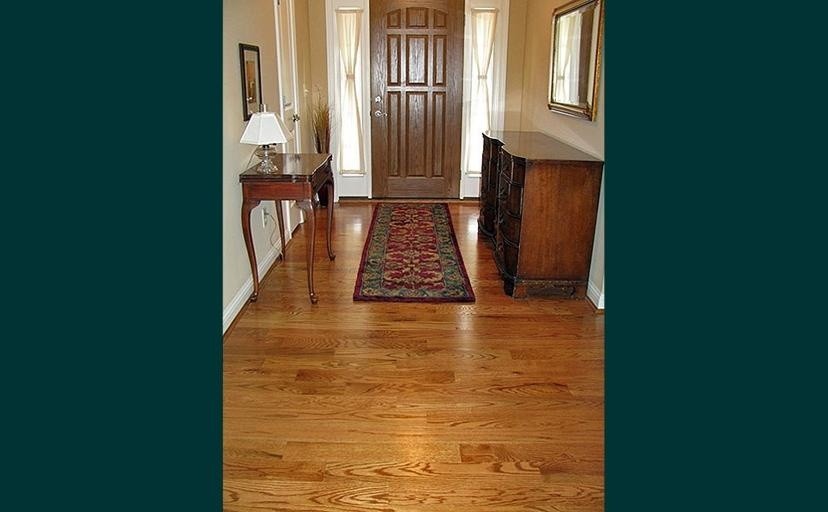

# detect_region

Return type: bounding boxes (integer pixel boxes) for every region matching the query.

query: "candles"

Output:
[311,97,334,209]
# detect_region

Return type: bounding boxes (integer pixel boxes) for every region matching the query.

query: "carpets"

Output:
[352,202,475,302]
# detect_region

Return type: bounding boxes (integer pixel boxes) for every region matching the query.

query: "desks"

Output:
[239,153,336,306]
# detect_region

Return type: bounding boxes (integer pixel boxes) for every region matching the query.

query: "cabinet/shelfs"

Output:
[477,131,604,299]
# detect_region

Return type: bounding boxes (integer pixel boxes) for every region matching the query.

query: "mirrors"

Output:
[547,0,604,122]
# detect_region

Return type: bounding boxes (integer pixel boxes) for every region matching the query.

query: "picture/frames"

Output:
[239,43,263,122]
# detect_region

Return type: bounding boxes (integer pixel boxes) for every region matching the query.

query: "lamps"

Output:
[238,112,294,175]
